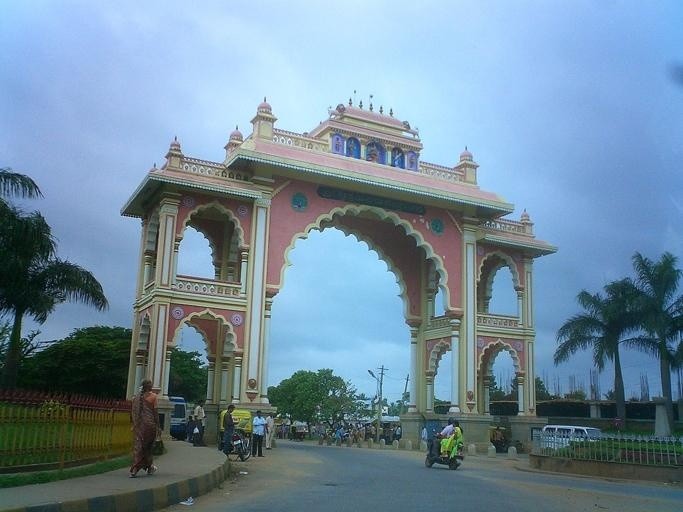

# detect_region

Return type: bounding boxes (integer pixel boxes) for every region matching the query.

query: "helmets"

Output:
[448,417,454,421]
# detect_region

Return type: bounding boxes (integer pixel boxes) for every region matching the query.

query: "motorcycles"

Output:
[420,426,467,472]
[231,424,251,464]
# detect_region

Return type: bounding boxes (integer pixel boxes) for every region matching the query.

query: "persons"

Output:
[495,427,504,453]
[265,412,275,449]
[193,400,207,447]
[440,421,464,459]
[335,421,401,443]
[432,417,454,458]
[281,423,288,438]
[222,405,238,461]
[421,426,427,441]
[129,379,162,478]
[252,411,268,457]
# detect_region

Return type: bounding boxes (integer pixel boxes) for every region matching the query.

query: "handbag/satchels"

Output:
[151,439,166,456]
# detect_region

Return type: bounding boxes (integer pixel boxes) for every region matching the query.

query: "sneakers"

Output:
[128,471,136,478]
[440,454,448,458]
[146,464,159,475]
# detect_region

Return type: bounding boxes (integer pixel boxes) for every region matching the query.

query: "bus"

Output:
[165,395,188,441]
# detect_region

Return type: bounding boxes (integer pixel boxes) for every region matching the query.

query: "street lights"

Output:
[366,369,382,440]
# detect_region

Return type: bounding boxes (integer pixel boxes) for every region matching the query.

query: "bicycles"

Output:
[492,440,524,453]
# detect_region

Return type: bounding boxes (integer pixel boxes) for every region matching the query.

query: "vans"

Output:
[537,424,609,457]
